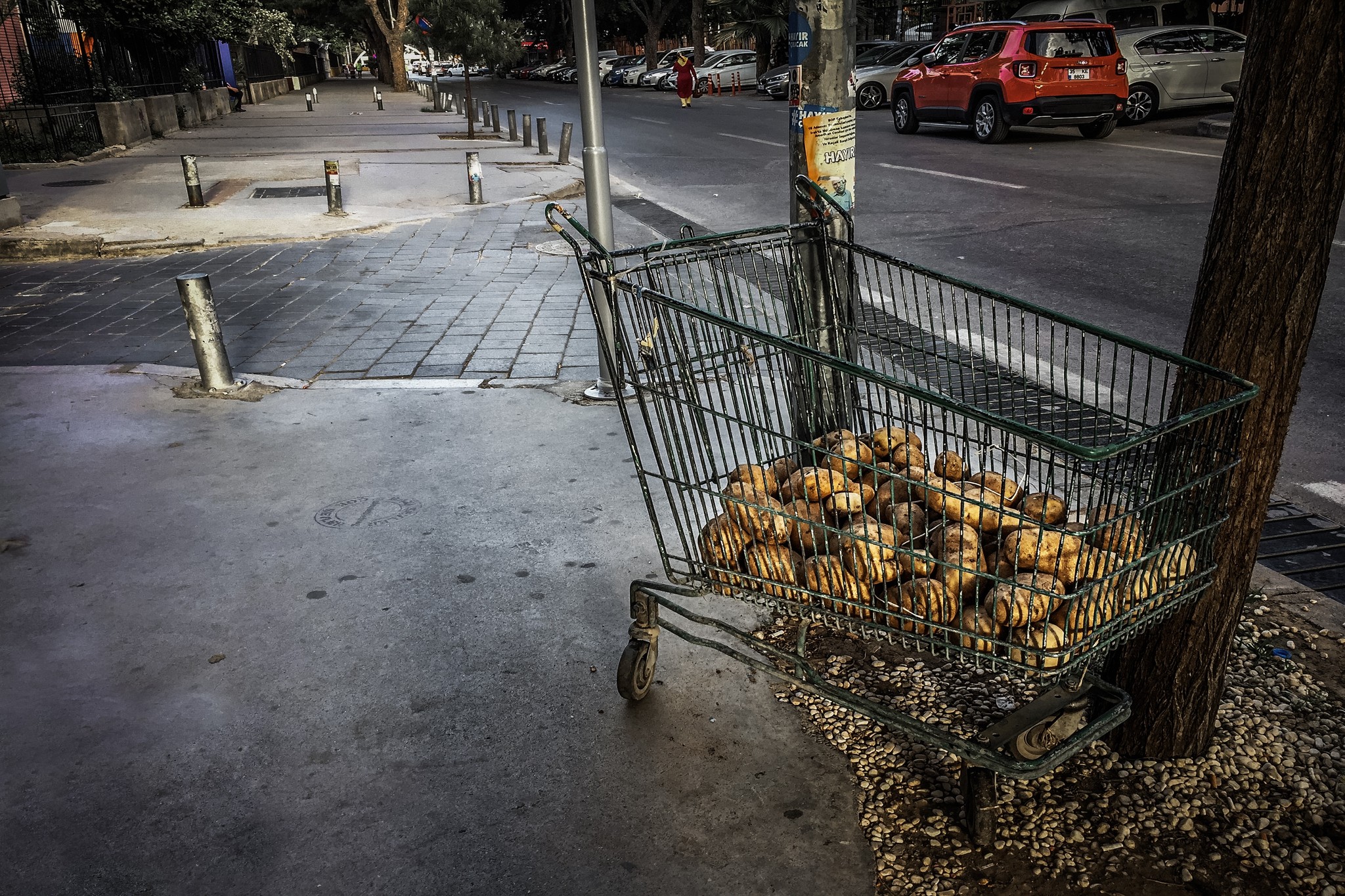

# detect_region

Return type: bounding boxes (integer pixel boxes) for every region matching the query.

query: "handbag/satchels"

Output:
[693,82,703,98]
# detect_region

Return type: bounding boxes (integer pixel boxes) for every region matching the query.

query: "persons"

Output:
[829,176,854,216]
[404,63,410,78]
[223,81,247,112]
[343,61,362,79]
[672,50,699,109]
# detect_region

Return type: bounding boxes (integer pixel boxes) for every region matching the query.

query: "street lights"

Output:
[345,40,362,65]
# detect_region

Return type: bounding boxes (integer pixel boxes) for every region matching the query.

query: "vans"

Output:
[1010,0,1214,49]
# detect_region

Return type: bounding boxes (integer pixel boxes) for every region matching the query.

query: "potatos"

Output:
[697,425,1198,679]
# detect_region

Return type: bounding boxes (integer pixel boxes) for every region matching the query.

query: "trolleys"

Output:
[545,174,1260,782]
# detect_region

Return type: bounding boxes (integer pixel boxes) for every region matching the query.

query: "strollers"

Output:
[350,69,356,79]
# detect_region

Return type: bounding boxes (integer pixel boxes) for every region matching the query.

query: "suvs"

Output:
[890,19,1129,143]
[608,46,715,88]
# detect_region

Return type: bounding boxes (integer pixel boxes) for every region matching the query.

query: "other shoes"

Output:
[358,77,360,79]
[234,107,246,112]
[682,106,686,109]
[687,103,691,107]
[360,77,362,78]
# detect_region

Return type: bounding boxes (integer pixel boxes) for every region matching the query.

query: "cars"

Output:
[665,49,757,93]
[509,56,577,82]
[905,23,961,42]
[412,61,429,76]
[439,62,454,75]
[640,51,712,92]
[447,63,492,77]
[1040,24,1247,125]
[553,50,640,86]
[426,63,444,77]
[757,39,938,111]
[361,65,370,71]
